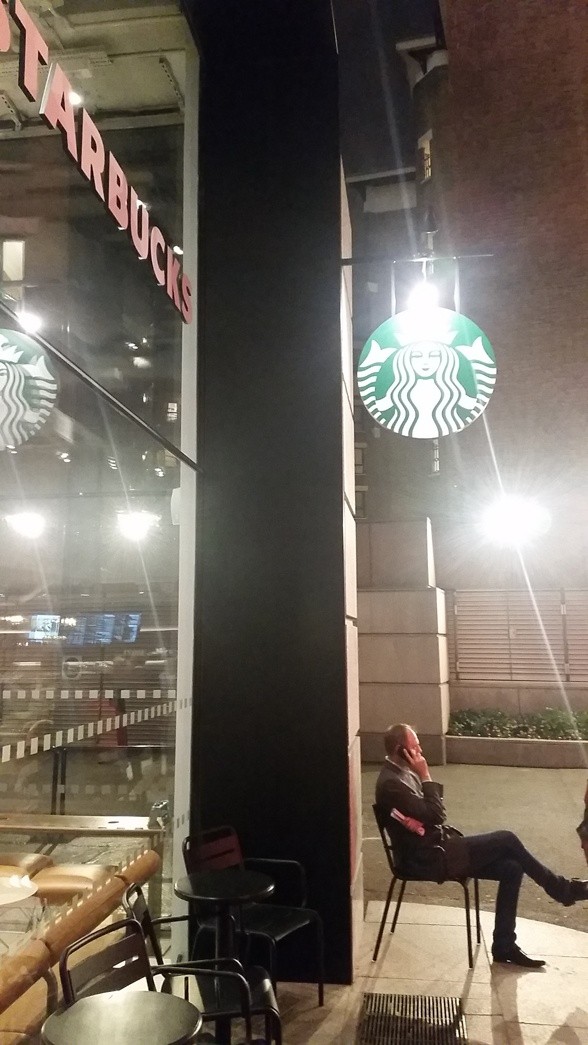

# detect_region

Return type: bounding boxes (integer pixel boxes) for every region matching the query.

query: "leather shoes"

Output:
[494,945,546,967]
[560,878,588,907]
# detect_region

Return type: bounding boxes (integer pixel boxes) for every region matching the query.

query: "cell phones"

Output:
[397,746,414,766]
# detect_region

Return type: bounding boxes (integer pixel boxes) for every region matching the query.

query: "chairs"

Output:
[58,825,323,1045]
[371,802,482,968]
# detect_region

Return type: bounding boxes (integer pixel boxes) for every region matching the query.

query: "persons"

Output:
[376,723,588,968]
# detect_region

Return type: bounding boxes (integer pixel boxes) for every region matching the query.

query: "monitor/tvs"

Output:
[64,610,142,645]
[28,613,62,642]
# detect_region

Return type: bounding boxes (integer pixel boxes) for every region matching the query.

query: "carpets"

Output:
[353,992,469,1045]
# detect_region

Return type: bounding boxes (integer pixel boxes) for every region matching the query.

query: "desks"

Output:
[0,877,39,949]
[0,813,175,931]
[40,989,202,1045]
[173,870,276,1045]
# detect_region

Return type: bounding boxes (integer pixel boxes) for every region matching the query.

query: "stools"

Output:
[30,862,118,914]
[0,851,53,879]
